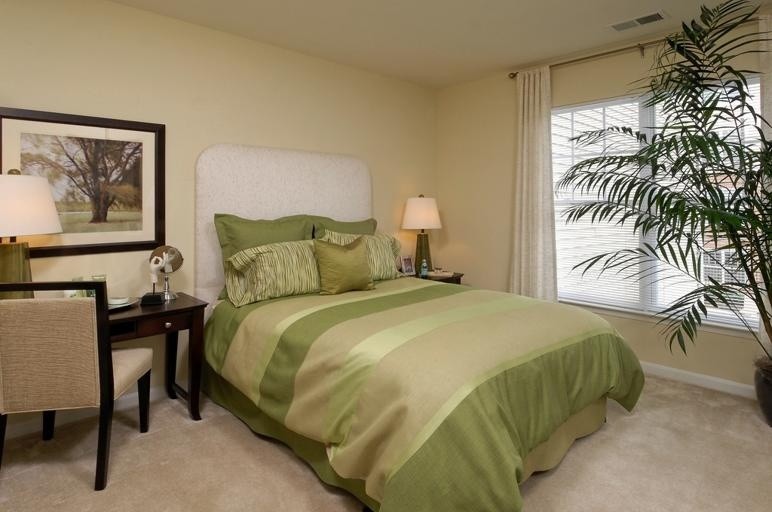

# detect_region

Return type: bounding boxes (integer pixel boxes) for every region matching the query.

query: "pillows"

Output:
[211,203,404,307]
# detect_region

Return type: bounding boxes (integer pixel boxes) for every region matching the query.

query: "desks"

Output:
[42,292,210,439]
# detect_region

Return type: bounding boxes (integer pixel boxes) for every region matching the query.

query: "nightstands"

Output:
[411,268,464,284]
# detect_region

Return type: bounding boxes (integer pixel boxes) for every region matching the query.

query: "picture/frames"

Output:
[0,108,166,261]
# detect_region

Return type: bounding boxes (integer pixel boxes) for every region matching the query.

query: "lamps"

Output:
[403,194,444,274]
[0,167,62,299]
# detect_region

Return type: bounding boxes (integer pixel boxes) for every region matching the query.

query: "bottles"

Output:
[421,259,427,275]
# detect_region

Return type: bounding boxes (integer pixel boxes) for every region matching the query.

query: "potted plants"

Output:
[554,9,770,430]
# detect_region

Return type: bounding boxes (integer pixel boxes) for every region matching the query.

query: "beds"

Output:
[193,146,648,509]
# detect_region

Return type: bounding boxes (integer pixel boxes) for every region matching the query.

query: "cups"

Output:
[434,264,449,272]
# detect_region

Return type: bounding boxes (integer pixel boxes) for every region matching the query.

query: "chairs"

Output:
[0,280,155,491]
